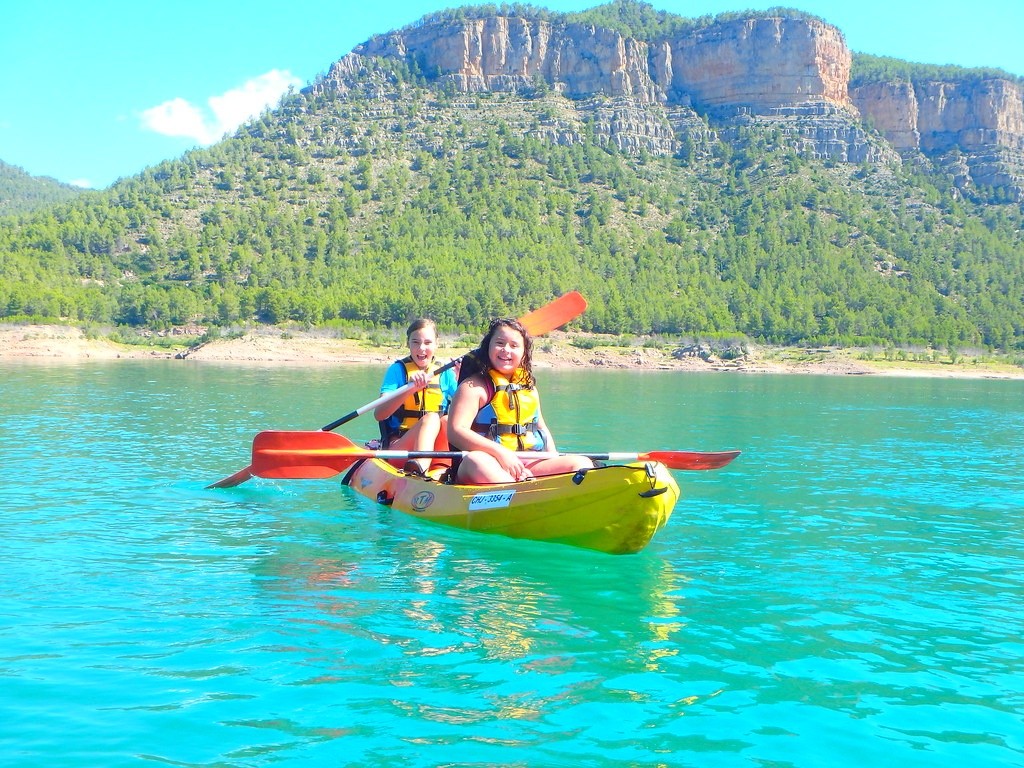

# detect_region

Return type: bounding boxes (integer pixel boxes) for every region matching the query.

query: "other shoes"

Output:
[403,456,432,477]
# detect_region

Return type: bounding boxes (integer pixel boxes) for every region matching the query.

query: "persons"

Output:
[374,319,463,477]
[447,318,594,482]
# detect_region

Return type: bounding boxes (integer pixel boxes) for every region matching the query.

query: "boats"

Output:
[345,438,681,555]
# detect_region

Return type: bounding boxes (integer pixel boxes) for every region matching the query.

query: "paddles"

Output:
[248,425,739,481]
[209,289,590,490]
